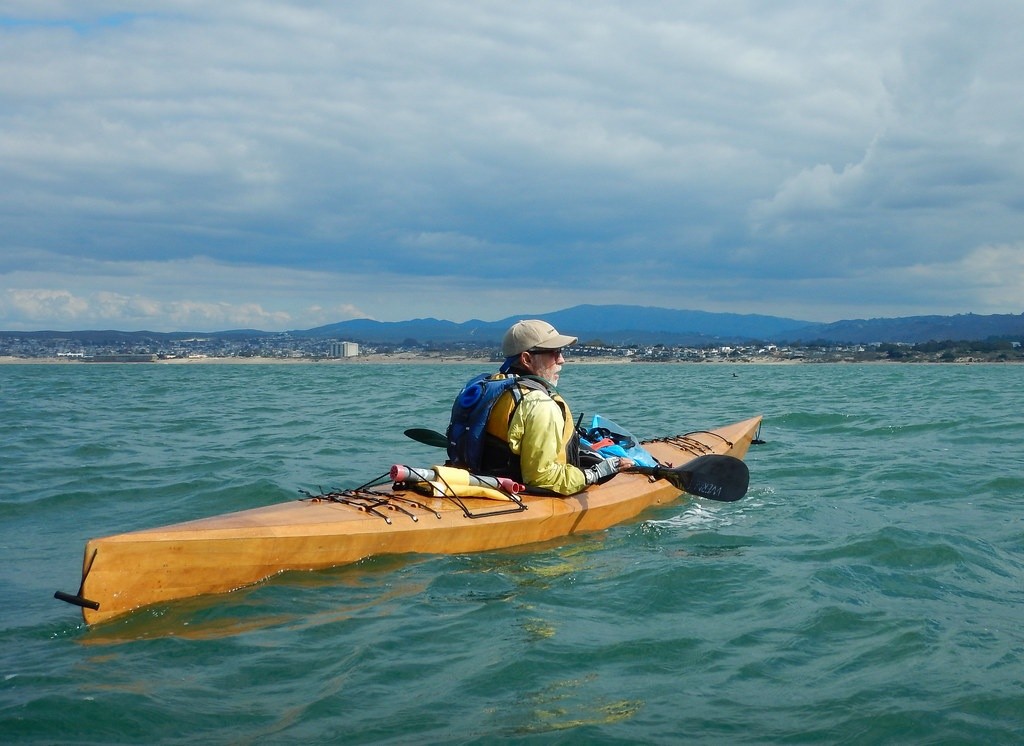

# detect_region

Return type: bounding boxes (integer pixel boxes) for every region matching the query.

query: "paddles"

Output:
[404,427,750,502]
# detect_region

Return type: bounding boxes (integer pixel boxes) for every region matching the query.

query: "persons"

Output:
[445,319,635,497]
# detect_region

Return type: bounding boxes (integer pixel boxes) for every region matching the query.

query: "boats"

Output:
[80,411,768,631]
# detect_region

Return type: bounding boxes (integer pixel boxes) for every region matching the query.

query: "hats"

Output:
[502,319,577,358]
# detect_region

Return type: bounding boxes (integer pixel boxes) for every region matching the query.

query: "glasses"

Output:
[528,348,561,354]
